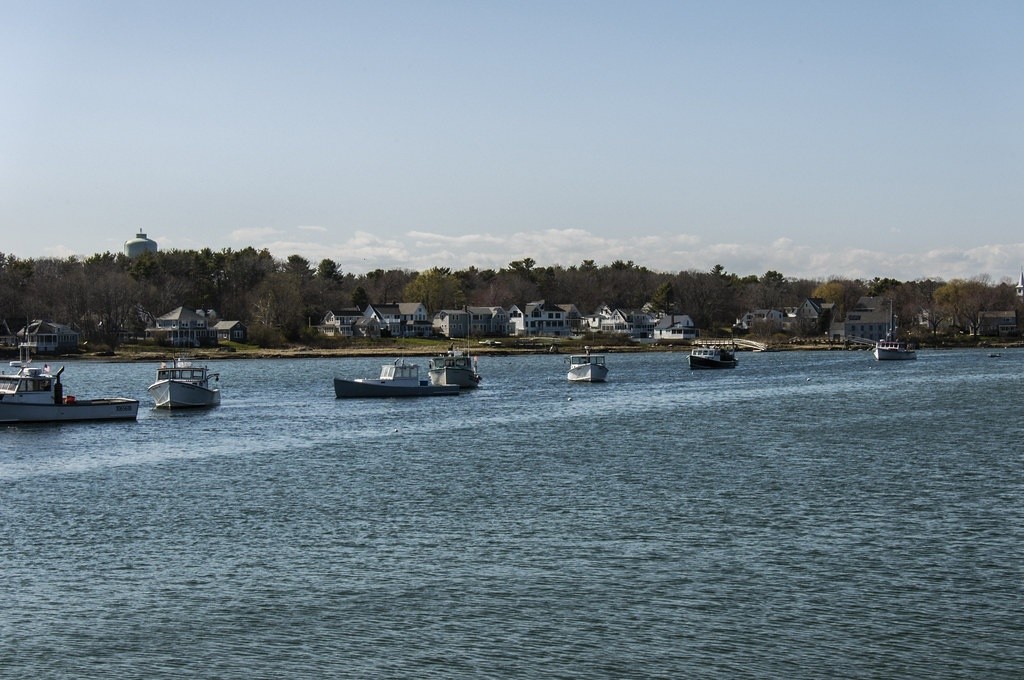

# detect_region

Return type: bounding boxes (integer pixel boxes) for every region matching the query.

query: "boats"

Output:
[566,344,609,382]
[987,354,1001,357]
[872,340,918,360]
[426,314,483,389]
[685,344,738,370]
[332,333,461,398]
[0,365,140,421]
[146,343,222,408]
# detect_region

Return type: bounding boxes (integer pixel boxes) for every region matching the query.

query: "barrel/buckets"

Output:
[420,381,428,386]
[67,395,75,403]
